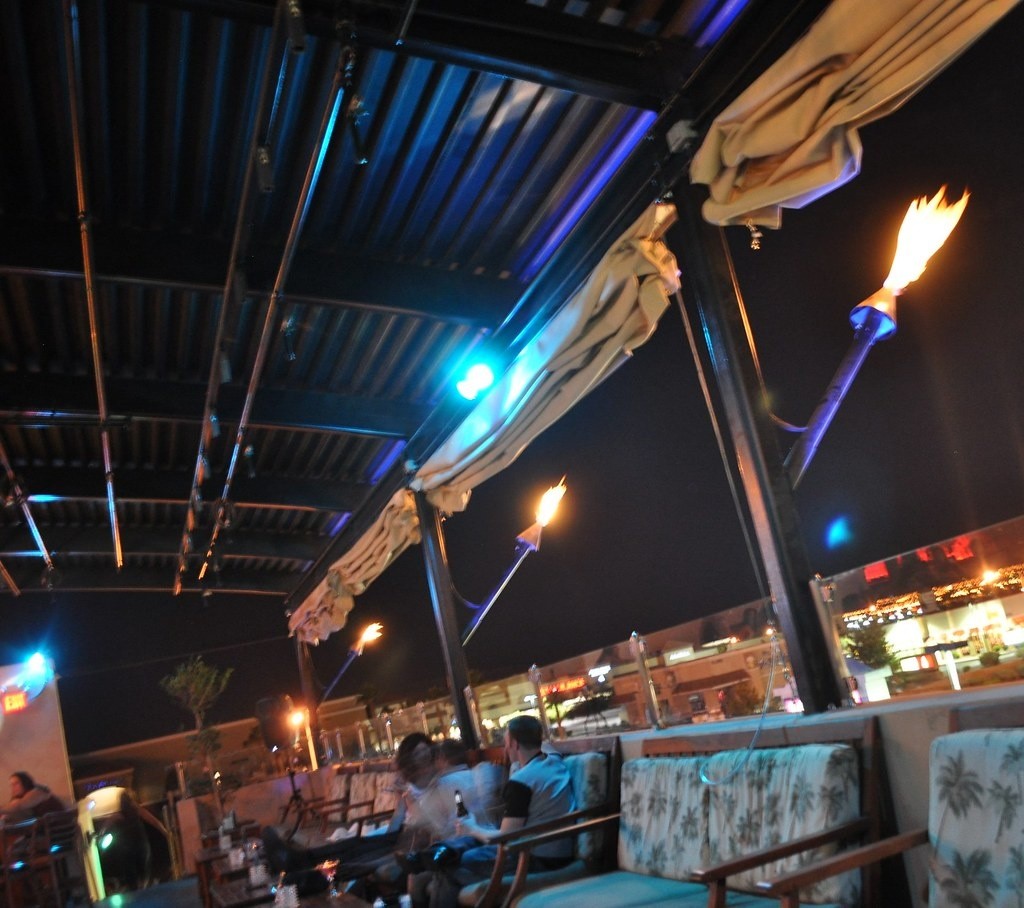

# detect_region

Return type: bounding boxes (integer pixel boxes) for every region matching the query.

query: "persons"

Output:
[0,771,79,862]
[333,715,575,908]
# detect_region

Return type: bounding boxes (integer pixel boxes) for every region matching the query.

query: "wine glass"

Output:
[317,859,340,902]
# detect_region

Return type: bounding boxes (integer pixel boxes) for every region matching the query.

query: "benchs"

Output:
[279,701,1024,908]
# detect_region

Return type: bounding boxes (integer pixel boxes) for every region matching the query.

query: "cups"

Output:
[249,864,267,885]
[219,835,232,851]
[228,848,244,866]
[276,884,300,908]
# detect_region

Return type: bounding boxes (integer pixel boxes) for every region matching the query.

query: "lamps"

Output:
[459,483,566,641]
[782,184,970,490]
[317,623,383,710]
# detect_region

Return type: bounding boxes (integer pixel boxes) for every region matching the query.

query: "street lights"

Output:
[320,687,482,760]
[810,573,863,704]
[529,664,551,739]
[630,632,666,729]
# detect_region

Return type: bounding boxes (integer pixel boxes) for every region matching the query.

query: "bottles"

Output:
[454,790,469,818]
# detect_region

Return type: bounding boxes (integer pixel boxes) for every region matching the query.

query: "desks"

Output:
[195,820,373,908]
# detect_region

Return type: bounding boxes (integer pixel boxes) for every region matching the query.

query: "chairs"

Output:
[0,808,91,908]
